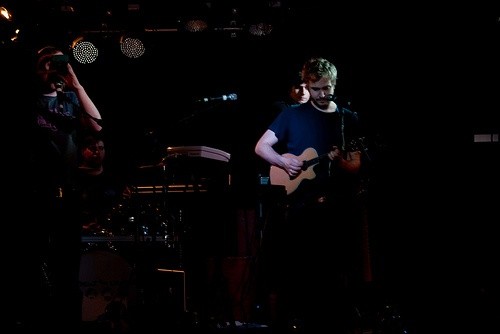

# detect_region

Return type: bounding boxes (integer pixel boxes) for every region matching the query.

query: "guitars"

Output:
[268,137,370,194]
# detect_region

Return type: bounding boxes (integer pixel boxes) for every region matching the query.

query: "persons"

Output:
[268,65,310,126]
[31,46,103,134]
[75,137,118,223]
[254,56,362,177]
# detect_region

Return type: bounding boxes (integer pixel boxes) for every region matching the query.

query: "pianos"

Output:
[167,146,231,163]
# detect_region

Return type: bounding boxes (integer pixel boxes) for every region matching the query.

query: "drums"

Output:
[78,248,137,334]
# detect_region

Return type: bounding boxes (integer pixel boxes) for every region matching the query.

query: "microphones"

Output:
[55,82,64,113]
[327,94,351,105]
[192,93,237,103]
